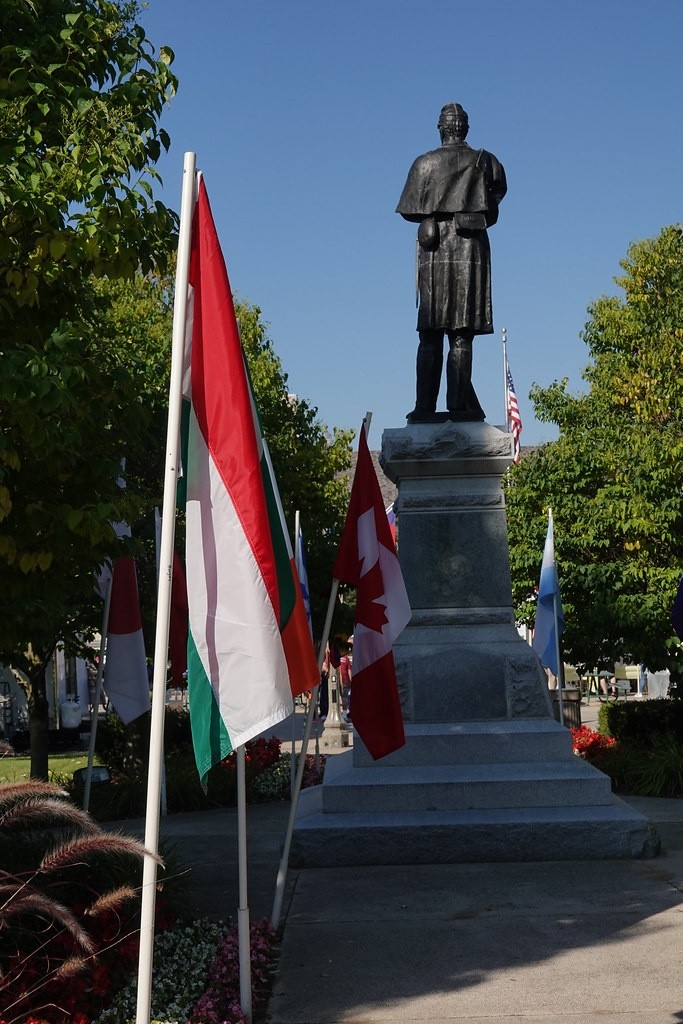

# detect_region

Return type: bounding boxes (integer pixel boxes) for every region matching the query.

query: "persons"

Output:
[543,654,648,703]
[395,103,507,422]
[300,636,354,723]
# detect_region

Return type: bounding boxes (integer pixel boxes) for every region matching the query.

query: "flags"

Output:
[297,525,313,646]
[531,516,566,689]
[386,505,395,548]
[507,362,523,473]
[526,585,539,602]
[94,453,152,727]
[332,418,414,761]
[183,179,320,796]
[0,666,29,711]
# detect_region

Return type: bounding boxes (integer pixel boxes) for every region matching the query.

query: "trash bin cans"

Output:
[72,766,112,823]
[549,689,582,729]
